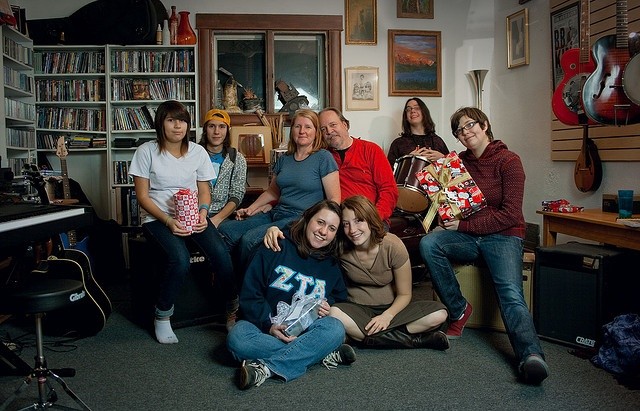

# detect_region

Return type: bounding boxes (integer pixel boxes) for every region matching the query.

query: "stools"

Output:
[0,277,92,411]
[434,252,534,333]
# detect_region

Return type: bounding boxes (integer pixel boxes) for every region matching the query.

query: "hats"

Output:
[204,109,230,127]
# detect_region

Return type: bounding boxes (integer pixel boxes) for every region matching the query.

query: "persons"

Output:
[225,197,357,391]
[386,96,453,239]
[319,107,397,232]
[567,19,577,46]
[418,108,550,387]
[222,109,341,263]
[127,103,240,344]
[197,108,248,229]
[325,195,450,352]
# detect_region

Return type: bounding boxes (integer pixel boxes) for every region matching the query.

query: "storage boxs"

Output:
[602,194,640,214]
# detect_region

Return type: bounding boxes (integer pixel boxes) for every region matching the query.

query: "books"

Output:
[32,52,106,73]
[110,77,195,101]
[35,106,106,132]
[5,96,37,123]
[8,158,29,175]
[37,131,107,149]
[30,153,38,172]
[36,80,106,102]
[111,49,195,72]
[616,218,640,228]
[111,187,141,227]
[111,105,157,131]
[113,161,134,185]
[5,127,37,149]
[4,65,34,94]
[186,105,196,129]
[2,35,34,69]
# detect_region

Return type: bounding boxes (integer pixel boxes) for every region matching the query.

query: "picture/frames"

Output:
[397,0,434,19]
[506,7,530,69]
[345,0,376,45]
[519,0,530,5]
[550,1,581,95]
[388,29,442,97]
[345,67,378,111]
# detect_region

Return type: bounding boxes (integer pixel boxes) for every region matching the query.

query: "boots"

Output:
[356,329,449,351]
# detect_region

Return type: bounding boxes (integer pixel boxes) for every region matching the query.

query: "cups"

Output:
[617,189,634,218]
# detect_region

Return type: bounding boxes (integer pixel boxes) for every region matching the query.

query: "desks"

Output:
[536,206,640,249]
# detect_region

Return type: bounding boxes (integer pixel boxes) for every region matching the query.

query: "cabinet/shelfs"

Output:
[106,43,200,226]
[33,45,111,221]
[0,23,37,201]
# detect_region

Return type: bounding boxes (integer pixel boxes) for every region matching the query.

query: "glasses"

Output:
[454,121,480,133]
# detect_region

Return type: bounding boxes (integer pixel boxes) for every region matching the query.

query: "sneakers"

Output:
[240,358,267,387]
[520,355,548,385]
[445,301,474,339]
[321,344,357,370]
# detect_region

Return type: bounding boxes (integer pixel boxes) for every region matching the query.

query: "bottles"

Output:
[169,5,178,45]
[163,20,171,46]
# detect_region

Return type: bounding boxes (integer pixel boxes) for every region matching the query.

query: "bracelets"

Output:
[163,216,172,227]
[198,204,210,211]
